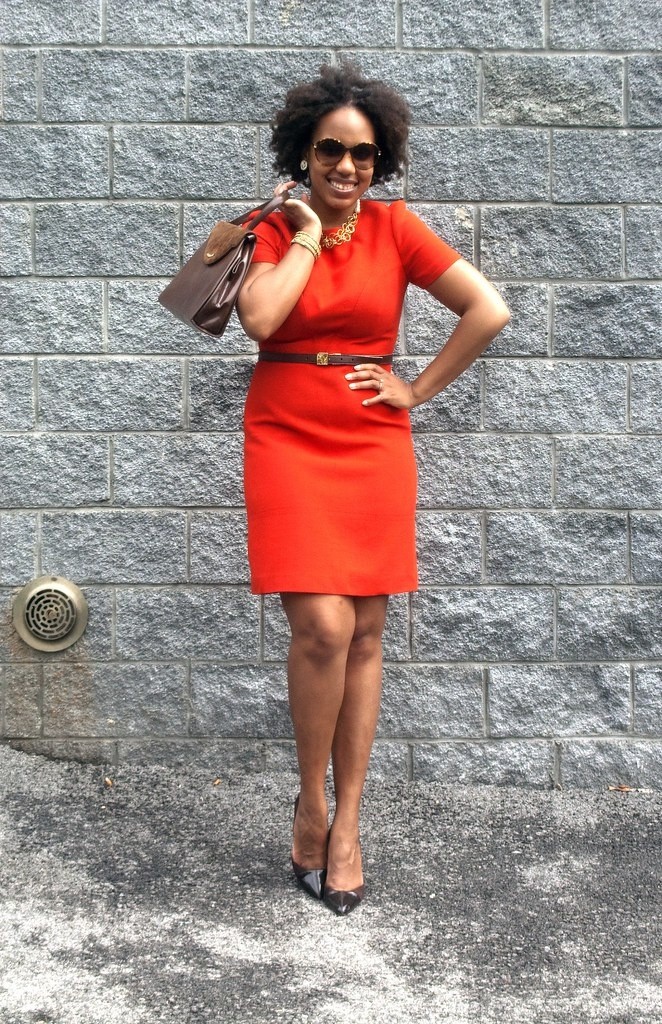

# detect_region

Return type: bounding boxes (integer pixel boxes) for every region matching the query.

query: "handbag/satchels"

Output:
[158,190,290,338]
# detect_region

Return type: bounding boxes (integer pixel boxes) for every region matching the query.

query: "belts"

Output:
[258,351,394,365]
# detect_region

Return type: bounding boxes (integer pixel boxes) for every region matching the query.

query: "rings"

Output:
[379,379,383,390]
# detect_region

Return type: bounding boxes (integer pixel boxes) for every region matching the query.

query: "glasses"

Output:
[312,138,381,170]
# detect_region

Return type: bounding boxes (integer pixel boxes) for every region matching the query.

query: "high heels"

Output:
[323,827,364,916]
[292,794,327,899]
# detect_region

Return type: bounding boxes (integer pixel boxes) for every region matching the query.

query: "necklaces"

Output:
[320,199,360,249]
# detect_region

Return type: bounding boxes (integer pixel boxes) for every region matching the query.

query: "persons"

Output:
[235,63,511,917]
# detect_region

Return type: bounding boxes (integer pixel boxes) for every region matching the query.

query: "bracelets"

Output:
[290,231,322,262]
